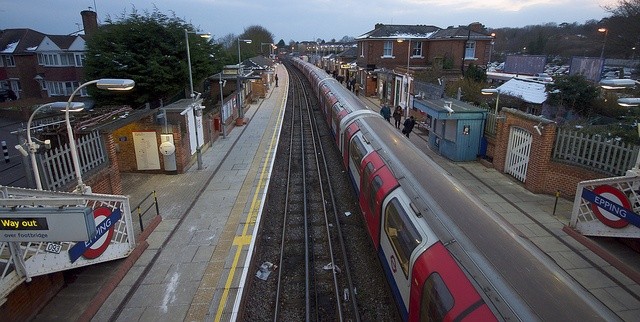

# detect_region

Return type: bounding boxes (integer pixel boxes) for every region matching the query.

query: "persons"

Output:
[380,103,391,123]
[323,65,359,96]
[393,106,402,129]
[275,73,279,87]
[403,116,415,138]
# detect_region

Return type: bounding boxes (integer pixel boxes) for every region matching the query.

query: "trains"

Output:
[290,57,605,322]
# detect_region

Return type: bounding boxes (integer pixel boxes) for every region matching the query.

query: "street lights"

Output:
[481,88,500,117]
[65,79,135,184]
[397,39,411,117]
[261,43,277,60]
[185,29,211,170]
[238,38,252,116]
[27,101,85,190]
